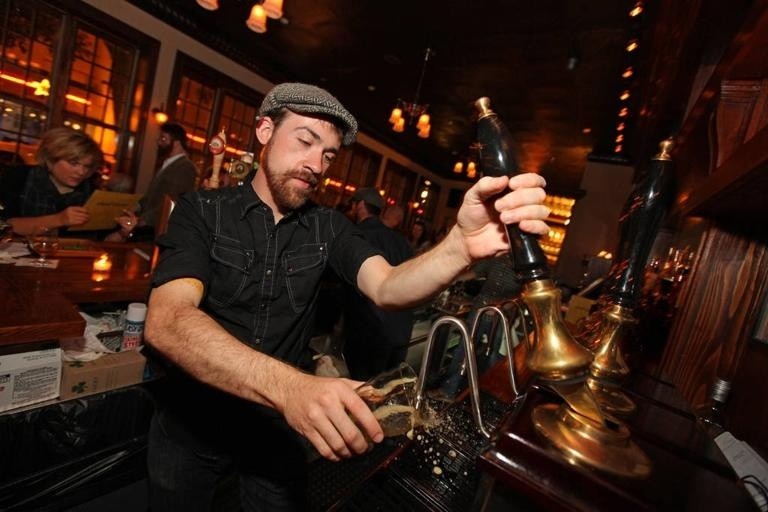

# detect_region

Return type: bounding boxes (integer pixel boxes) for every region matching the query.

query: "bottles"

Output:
[119,302,148,353]
[691,378,731,446]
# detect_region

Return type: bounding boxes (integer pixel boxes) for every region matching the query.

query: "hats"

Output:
[352,187,386,208]
[260,82,359,146]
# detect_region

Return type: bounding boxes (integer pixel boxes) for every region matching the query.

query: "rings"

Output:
[127,220,131,225]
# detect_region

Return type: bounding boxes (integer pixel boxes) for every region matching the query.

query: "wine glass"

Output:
[27,222,60,268]
[0,222,17,264]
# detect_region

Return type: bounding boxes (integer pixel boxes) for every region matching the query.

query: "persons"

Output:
[136,122,196,243]
[0,128,139,242]
[142,82,552,512]
[336,189,449,382]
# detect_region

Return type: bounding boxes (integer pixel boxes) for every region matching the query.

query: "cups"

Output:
[345,362,429,443]
